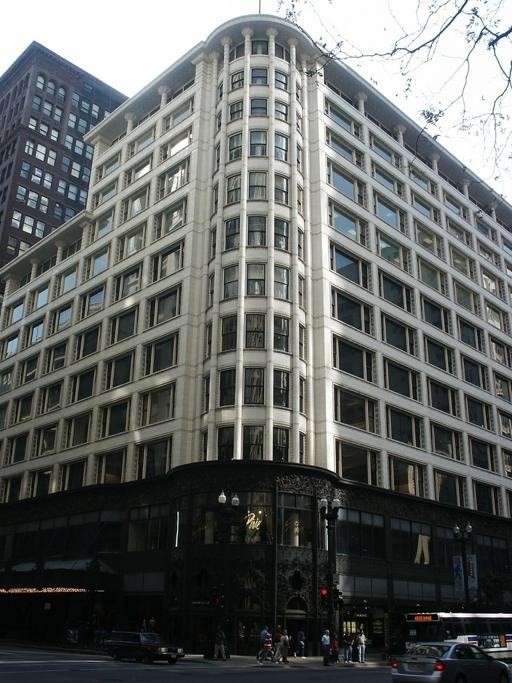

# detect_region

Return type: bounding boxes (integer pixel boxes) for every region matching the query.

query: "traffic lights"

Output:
[320,585,344,610]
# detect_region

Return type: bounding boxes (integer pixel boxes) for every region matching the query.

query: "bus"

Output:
[400,610,512,662]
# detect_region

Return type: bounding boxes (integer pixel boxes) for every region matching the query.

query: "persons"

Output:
[211,624,228,662]
[255,623,369,667]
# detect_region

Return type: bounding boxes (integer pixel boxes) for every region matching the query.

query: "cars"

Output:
[390,641,511,682]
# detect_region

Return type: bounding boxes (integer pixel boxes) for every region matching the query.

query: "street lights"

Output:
[216,487,242,656]
[319,497,341,633]
[452,521,472,608]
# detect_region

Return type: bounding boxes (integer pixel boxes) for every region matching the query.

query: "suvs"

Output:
[104,628,185,665]
